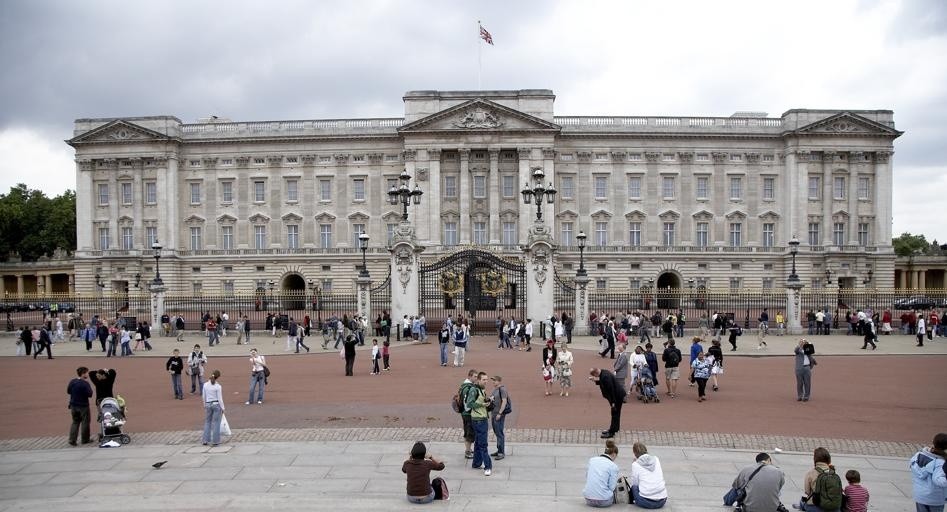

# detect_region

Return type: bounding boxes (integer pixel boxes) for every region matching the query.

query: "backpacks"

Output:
[815,466,844,510]
[299,327,306,337]
[432,477,450,500]
[376,350,382,359]
[452,385,467,412]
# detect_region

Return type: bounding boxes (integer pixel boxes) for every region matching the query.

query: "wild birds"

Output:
[152,461,168,469]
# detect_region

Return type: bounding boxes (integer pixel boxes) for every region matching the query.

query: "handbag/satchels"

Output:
[804,342,815,354]
[612,476,635,505]
[341,342,347,358]
[499,386,512,415]
[725,489,738,507]
[264,367,270,377]
[219,410,231,436]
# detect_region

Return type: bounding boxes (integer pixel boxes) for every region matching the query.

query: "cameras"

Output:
[485,398,495,411]
[803,339,806,342]
[351,335,354,336]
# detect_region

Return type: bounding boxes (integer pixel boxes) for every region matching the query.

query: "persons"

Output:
[910,433,947,512]
[402,442,445,504]
[792,448,842,512]
[630,443,667,508]
[583,440,622,508]
[723,453,785,512]
[842,471,869,512]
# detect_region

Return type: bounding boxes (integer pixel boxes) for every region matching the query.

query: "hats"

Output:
[546,340,554,346]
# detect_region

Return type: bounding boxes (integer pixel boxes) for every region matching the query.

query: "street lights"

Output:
[386,168,424,221]
[150,239,164,284]
[575,229,589,277]
[787,234,802,281]
[687,277,693,299]
[358,230,371,276]
[520,168,558,225]
[268,280,274,299]
[307,278,314,308]
[649,277,654,294]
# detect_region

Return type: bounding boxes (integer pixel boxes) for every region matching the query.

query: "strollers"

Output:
[635,365,661,405]
[96,395,132,448]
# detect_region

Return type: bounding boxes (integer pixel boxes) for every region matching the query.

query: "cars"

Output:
[0,298,76,314]
[894,296,947,311]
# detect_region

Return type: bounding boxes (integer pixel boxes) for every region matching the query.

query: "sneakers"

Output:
[496,341,532,351]
[478,463,485,469]
[200,393,202,395]
[545,391,569,397]
[665,391,677,397]
[798,396,809,401]
[190,390,197,393]
[689,382,696,386]
[85,348,150,357]
[203,441,222,446]
[440,362,465,368]
[713,385,721,390]
[495,455,505,460]
[175,397,184,400]
[244,400,262,405]
[69,438,93,446]
[492,452,499,456]
[699,396,707,401]
[484,467,492,476]
[465,452,474,459]
[208,341,250,346]
[464,449,474,452]
[16,351,55,360]
